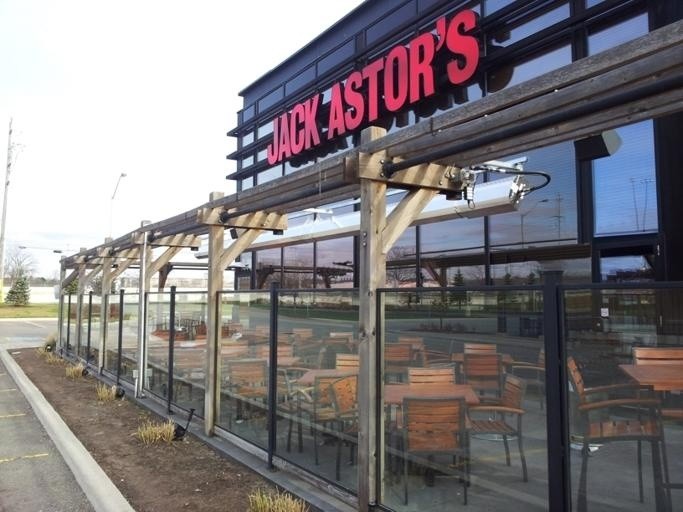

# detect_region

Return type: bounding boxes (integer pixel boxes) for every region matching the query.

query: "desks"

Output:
[619,360,682,511]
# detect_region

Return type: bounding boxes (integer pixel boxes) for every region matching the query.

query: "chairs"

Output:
[632,347,682,400]
[510,346,546,410]
[154,325,313,423]
[567,356,671,511]
[278,331,528,505]
